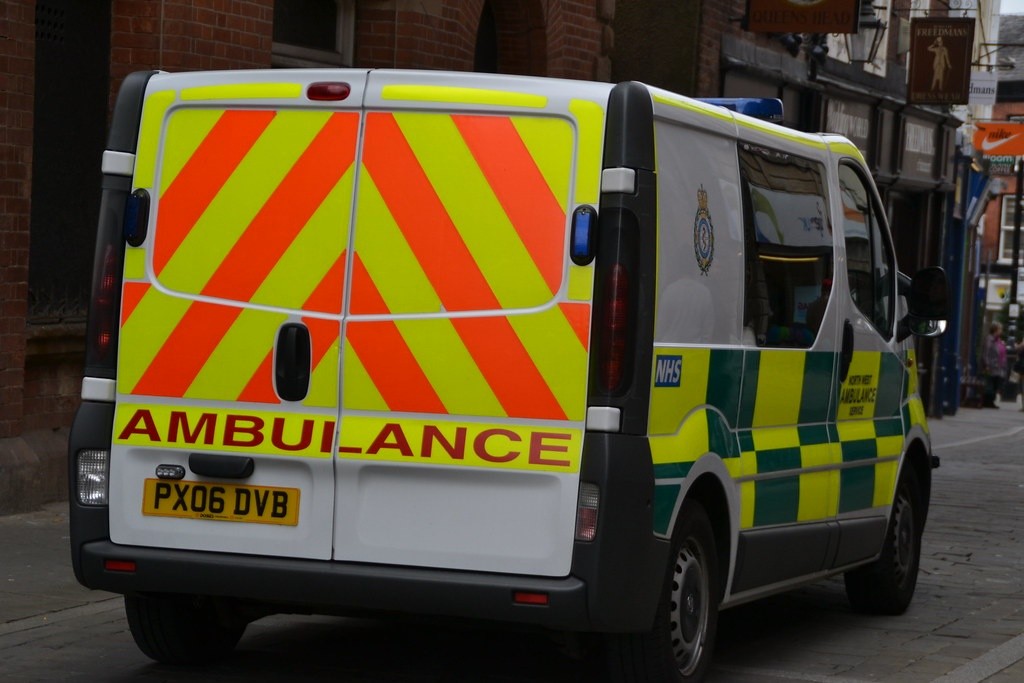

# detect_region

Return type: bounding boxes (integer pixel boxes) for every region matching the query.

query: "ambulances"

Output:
[67,65,947,683]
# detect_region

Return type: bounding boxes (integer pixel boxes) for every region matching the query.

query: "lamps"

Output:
[844,0,888,62]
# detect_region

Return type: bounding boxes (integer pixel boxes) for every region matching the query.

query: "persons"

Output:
[1014,306,1024,412]
[982,320,1006,410]
